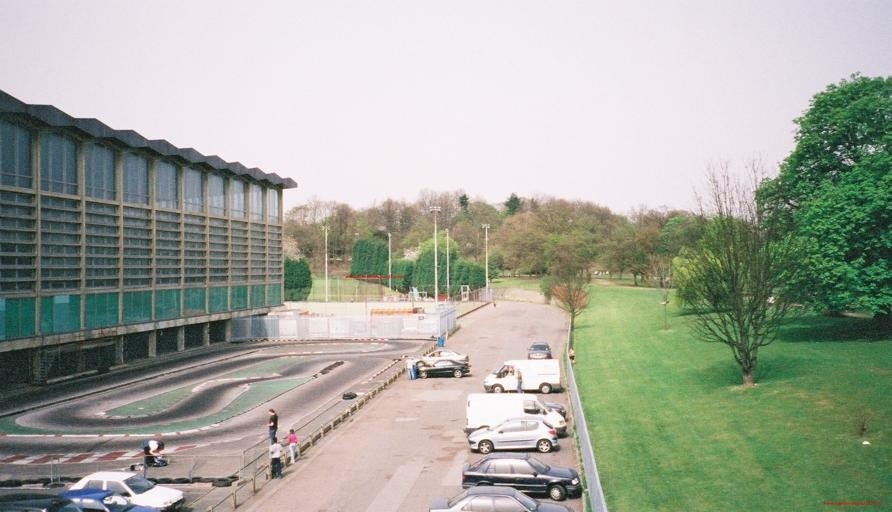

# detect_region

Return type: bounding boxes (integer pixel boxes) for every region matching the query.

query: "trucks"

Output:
[464,391,567,437]
[483,359,561,392]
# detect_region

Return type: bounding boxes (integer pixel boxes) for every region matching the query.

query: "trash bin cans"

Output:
[438,336,443,347]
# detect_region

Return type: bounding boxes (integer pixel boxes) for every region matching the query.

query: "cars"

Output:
[462,452,583,501]
[413,349,471,366]
[540,401,567,417]
[418,359,470,379]
[59,486,162,512]
[467,416,560,452]
[0,487,81,511]
[527,341,551,359]
[429,486,575,511]
[65,470,186,512]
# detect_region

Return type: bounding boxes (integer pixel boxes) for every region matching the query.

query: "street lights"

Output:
[320,224,331,301]
[387,231,393,297]
[445,228,450,301]
[430,206,443,304]
[482,223,491,302]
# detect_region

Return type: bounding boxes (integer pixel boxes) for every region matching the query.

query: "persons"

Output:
[266,408,277,444]
[270,438,284,478]
[407,357,415,381]
[288,428,298,464]
[516,368,524,393]
[569,347,575,369]
[144,440,168,467]
